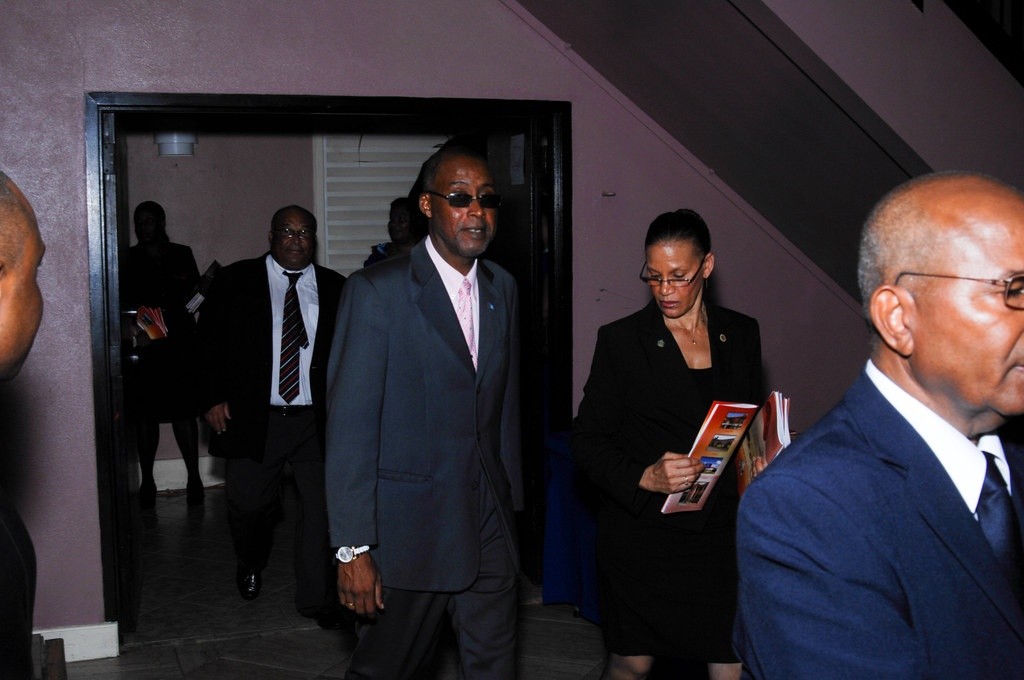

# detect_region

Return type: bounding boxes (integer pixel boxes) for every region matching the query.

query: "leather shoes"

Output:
[235,563,262,600]
[313,607,346,631]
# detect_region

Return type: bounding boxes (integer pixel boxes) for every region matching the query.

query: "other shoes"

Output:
[141,476,157,493]
[186,477,205,497]
[519,590,544,607]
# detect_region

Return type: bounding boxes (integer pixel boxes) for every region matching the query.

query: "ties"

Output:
[279,271,309,403]
[977,450,1024,614]
[456,278,478,376]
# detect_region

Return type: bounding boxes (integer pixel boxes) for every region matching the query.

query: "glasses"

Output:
[640,256,704,288]
[278,228,313,240]
[894,273,1023,312]
[428,189,502,209]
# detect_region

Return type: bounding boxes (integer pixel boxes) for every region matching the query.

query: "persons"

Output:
[371,197,417,262]
[324,148,524,680]
[0,168,46,680]
[121,324,138,340]
[567,210,764,680]
[733,171,1024,680]
[195,206,345,631]
[127,201,205,508]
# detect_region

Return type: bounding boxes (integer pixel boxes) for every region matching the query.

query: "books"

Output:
[128,305,169,347]
[661,400,757,514]
[736,390,791,497]
[186,261,220,314]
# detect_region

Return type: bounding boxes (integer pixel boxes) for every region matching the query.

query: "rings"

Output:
[347,603,354,606]
[685,476,688,485]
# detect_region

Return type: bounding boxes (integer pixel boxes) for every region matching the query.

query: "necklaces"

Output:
[690,335,696,344]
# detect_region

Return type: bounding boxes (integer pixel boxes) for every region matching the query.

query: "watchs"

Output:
[335,545,370,563]
